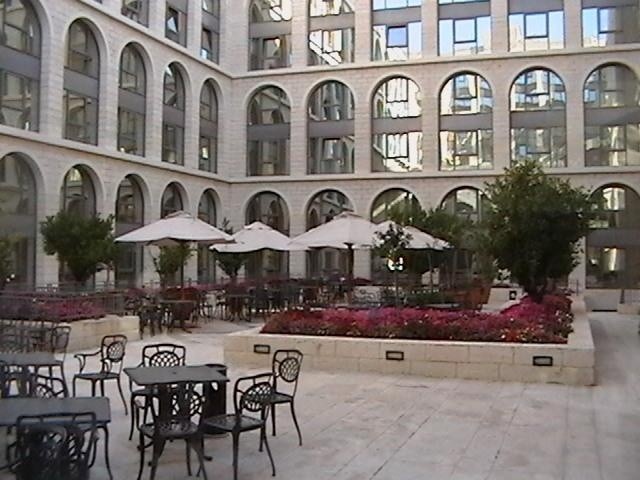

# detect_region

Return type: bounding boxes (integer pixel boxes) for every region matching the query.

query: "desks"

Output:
[119,365,232,466]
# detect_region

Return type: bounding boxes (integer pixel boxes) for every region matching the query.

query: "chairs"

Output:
[134,379,218,480]
[104,273,356,340]
[124,342,195,440]
[237,347,307,452]
[0,316,130,480]
[191,369,280,480]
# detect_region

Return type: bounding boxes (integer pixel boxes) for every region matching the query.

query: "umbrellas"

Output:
[111,208,455,311]
